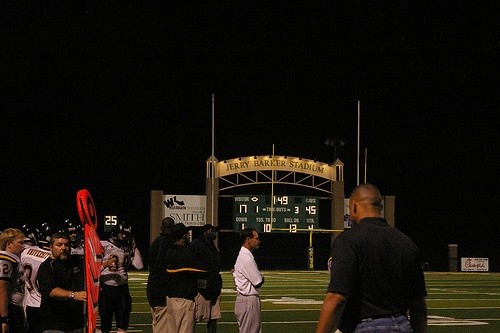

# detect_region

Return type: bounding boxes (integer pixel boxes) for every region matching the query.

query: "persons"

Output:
[188,224,223,333]
[21,223,52,333]
[0,227,29,333]
[99,222,143,333]
[35,232,87,333]
[233,227,265,333]
[62,218,84,257]
[145,217,175,333]
[316,183,428,333]
[166,222,210,333]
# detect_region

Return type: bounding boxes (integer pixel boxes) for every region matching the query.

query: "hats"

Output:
[200,224,222,236]
[172,223,192,238]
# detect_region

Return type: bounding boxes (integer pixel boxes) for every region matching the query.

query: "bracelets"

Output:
[1,315,10,323]
[70,291,75,300]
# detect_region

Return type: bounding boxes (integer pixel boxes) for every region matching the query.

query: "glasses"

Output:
[67,230,77,235]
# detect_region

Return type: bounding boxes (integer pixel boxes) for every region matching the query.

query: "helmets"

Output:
[111,222,131,236]
[21,224,39,245]
[38,223,51,243]
[61,218,82,249]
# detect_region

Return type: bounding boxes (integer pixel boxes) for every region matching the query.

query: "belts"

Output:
[363,310,404,320]
